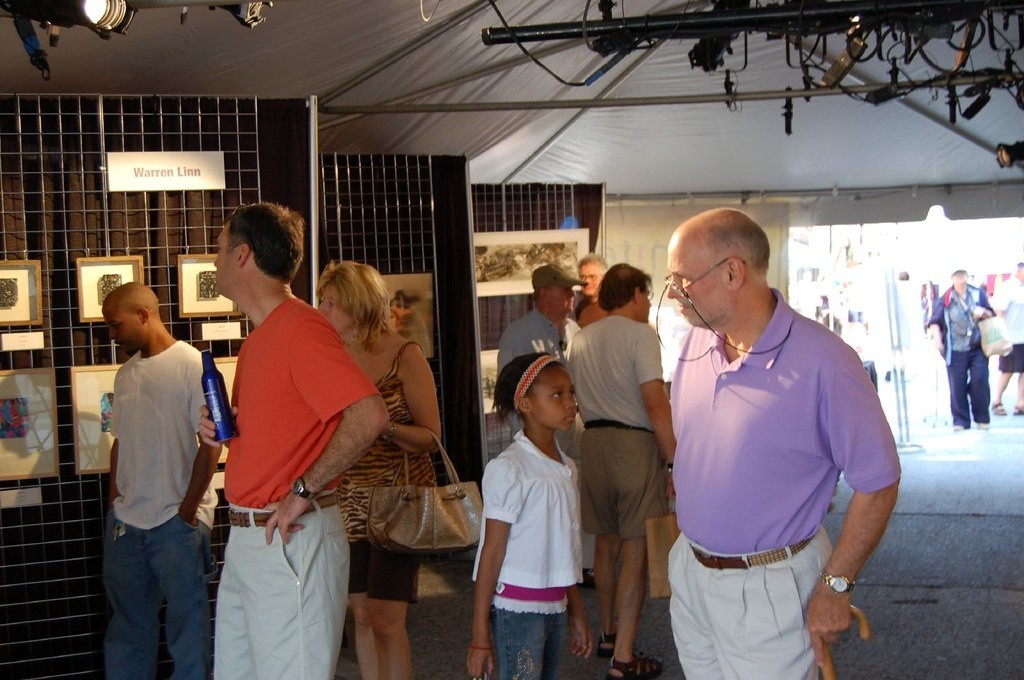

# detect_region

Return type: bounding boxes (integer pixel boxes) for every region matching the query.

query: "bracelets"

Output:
[468,645,493,650]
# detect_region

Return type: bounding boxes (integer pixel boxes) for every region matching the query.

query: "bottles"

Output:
[201,350,237,442]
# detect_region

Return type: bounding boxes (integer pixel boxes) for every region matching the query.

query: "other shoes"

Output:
[978,422,988,430]
[953,425,965,431]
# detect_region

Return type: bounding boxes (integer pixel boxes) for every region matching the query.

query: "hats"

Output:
[949,267,967,276]
[532,264,590,289]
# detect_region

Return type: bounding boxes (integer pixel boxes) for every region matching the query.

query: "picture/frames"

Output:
[0,260,43,326]
[0,368,59,481]
[177,254,242,318]
[480,349,500,413]
[71,365,122,474]
[473,228,589,296]
[76,254,144,323]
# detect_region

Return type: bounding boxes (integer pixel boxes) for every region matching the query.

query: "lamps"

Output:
[689,0,751,74]
[995,140,1024,167]
[81,0,137,35]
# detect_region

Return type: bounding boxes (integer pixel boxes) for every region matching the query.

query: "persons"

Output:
[465,352,594,680]
[315,258,441,679]
[921,266,995,431]
[567,253,606,328]
[990,261,1024,416]
[667,207,902,680]
[497,264,595,590]
[197,202,390,680]
[101,282,224,680]
[568,264,677,680]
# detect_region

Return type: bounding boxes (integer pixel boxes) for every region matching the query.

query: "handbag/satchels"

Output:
[646,495,682,600]
[366,429,485,552]
[979,311,1014,357]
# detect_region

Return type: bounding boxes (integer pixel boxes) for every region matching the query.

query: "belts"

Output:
[691,536,812,570]
[227,493,337,526]
[583,418,647,430]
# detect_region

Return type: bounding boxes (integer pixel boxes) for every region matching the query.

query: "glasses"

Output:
[665,257,747,300]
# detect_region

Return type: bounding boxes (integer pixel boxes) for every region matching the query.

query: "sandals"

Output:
[1013,408,1024,416]
[992,402,1006,416]
[606,652,664,680]
[597,632,616,658]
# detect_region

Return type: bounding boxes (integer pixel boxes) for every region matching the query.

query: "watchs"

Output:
[661,461,673,473]
[378,422,400,443]
[291,477,317,500]
[818,569,855,594]
